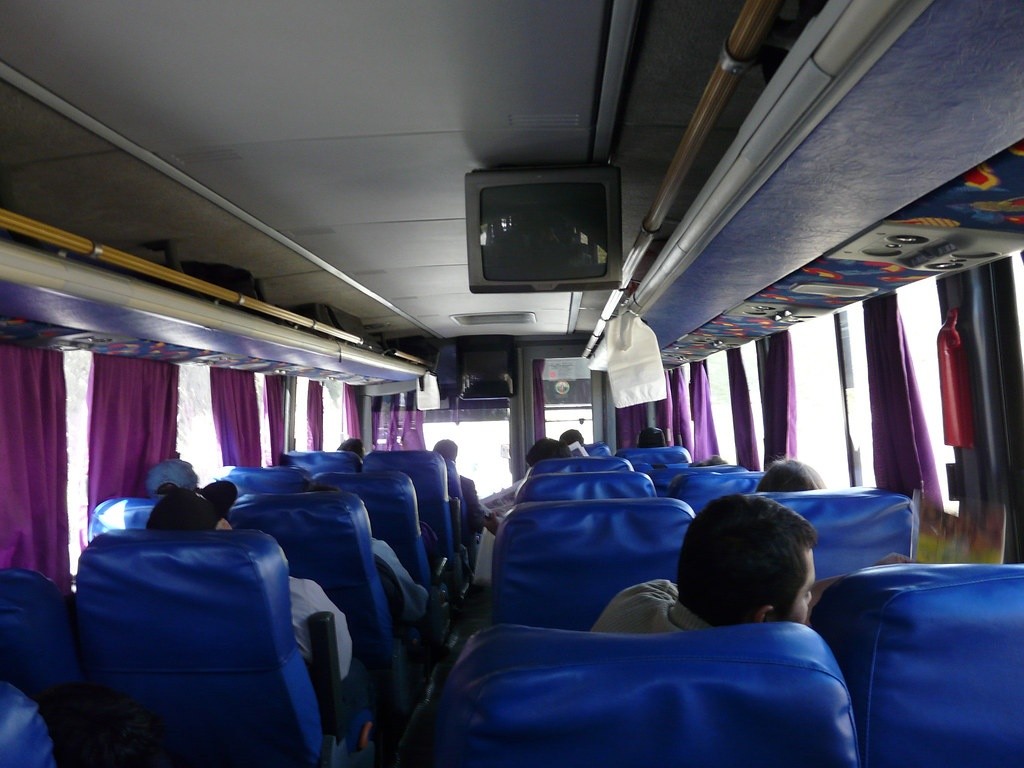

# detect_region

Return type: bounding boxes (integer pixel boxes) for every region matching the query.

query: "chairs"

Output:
[0,442,1024,768]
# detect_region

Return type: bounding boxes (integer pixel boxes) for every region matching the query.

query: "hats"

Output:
[146,480,238,532]
[144,458,198,495]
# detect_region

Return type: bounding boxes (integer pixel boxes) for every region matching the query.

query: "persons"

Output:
[485,429,585,535]
[307,484,432,626]
[147,480,373,768]
[638,426,667,447]
[667,455,727,497]
[338,437,364,459]
[755,458,827,493]
[433,439,487,537]
[588,497,919,635]
[144,457,196,498]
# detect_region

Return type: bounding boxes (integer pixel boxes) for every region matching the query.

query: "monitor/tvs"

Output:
[459,344,520,400]
[465,166,623,293]
